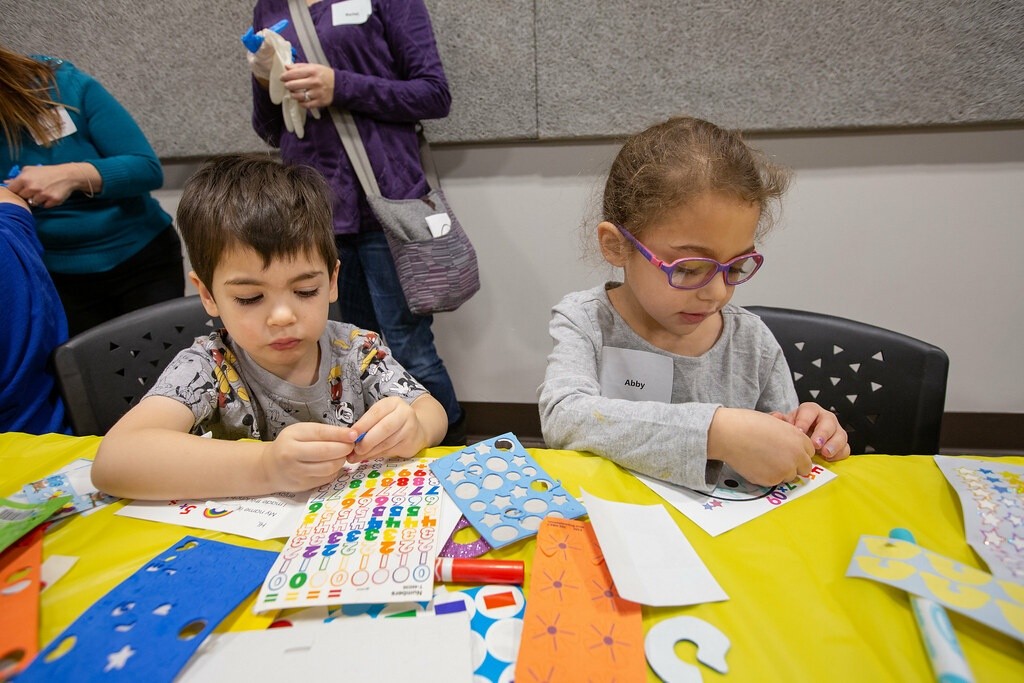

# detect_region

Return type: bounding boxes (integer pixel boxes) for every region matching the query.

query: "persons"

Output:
[91,156,449,500]
[250,0,468,448]
[0,46,185,314]
[536,119,851,492]
[0,178,74,436]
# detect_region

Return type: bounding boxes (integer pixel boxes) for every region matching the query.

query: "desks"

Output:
[0,415,1024,682]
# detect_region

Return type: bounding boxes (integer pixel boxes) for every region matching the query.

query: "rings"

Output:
[304,90,310,104]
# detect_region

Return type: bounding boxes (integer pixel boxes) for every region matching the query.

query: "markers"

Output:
[889,528,972,682]
[434,556,525,585]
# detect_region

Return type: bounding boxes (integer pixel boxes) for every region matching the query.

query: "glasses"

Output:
[608,217,762,292]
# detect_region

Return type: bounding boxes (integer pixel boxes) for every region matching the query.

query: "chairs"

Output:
[56,289,221,437]
[732,304,950,455]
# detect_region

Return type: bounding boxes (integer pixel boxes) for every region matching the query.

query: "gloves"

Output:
[243,18,322,138]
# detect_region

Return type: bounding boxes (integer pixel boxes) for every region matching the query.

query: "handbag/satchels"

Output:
[366,190,480,317]
